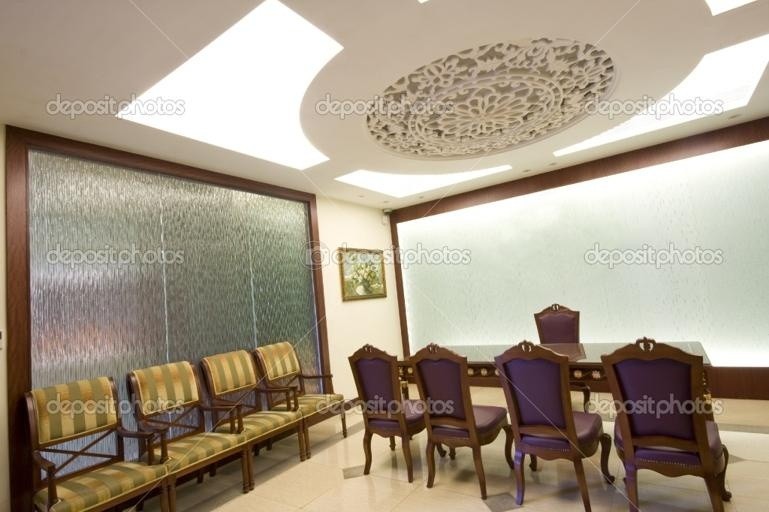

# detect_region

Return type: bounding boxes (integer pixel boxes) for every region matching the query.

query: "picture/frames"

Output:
[339,247,387,301]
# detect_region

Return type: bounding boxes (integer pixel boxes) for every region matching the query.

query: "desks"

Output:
[399,342,712,460]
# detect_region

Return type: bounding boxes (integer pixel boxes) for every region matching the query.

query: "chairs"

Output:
[201,351,305,490]
[535,304,591,414]
[602,336,732,511]
[348,343,447,483]
[127,361,250,511]
[254,341,347,458]
[17,374,170,512]
[410,342,515,499]
[494,340,614,512]
